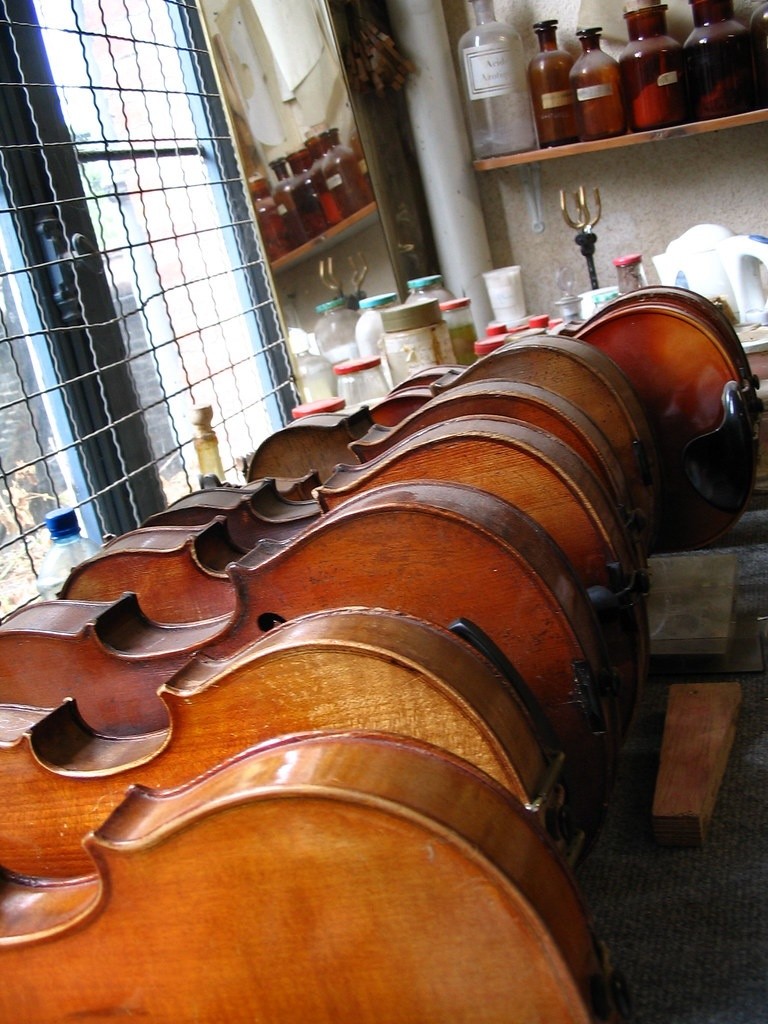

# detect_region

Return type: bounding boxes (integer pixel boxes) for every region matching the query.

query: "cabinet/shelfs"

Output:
[471,109,767,174]
[268,200,377,274]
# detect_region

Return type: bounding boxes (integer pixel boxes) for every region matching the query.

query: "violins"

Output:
[1,287,759,1021]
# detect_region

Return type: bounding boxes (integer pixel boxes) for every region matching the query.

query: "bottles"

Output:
[355,292,397,391]
[613,250,648,295]
[247,129,376,261]
[37,506,106,601]
[458,0,536,160]
[527,19,578,148]
[619,0,688,131]
[290,339,336,401]
[682,0,758,123]
[314,297,361,364]
[404,274,457,309]
[567,25,626,140]
[333,356,390,407]
[748,0,768,110]
[191,405,225,485]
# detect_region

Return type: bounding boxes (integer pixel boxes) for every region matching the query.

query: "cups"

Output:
[483,265,526,322]
